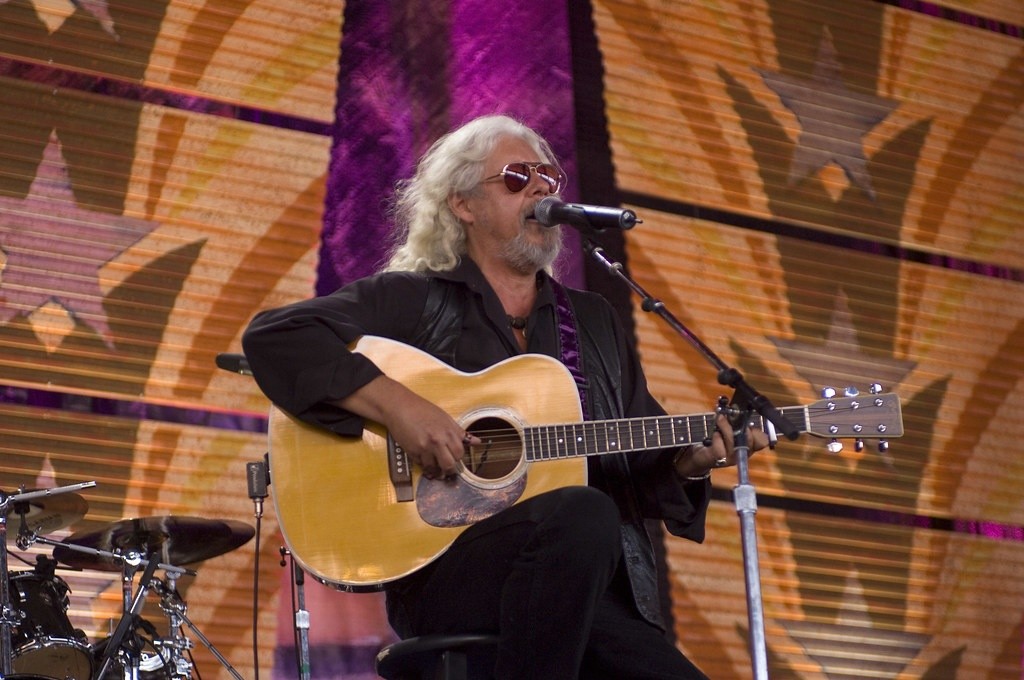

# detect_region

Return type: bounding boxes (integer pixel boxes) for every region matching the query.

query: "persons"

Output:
[241,115,770,679]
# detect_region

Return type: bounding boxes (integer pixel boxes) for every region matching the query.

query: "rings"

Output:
[463,430,473,445]
[715,458,727,467]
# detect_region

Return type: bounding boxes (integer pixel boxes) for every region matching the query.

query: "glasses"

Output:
[477,161,560,195]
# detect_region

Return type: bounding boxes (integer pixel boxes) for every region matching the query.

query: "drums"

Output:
[1,569,93,678]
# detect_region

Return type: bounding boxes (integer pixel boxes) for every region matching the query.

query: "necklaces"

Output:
[507,314,532,338]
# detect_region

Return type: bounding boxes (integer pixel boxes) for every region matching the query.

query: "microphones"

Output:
[215,353,254,376]
[534,195,643,230]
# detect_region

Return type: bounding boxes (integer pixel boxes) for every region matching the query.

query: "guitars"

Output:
[267,335,905,594]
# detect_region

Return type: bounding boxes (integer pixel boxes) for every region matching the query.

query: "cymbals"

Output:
[5,489,88,546]
[52,515,256,571]
